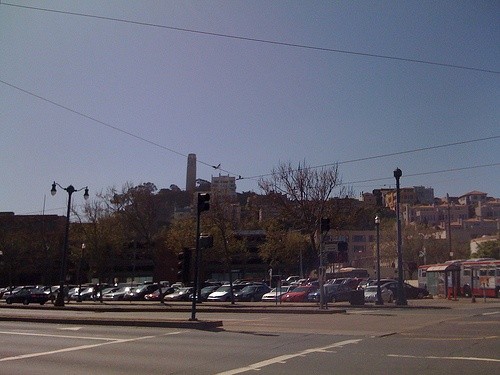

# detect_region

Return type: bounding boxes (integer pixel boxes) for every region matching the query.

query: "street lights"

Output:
[374,216,385,304]
[392,166,408,305]
[49,181,89,307]
[77,242,86,303]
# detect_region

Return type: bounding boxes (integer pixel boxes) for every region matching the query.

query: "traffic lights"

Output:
[319,216,330,233]
[175,248,190,284]
[198,192,212,214]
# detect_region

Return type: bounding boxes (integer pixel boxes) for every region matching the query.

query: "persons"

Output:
[92,284,99,301]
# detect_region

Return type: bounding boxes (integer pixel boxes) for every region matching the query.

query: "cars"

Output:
[6,289,50,306]
[0,268,429,311]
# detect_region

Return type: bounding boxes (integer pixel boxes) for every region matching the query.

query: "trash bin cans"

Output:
[349,290,364,304]
